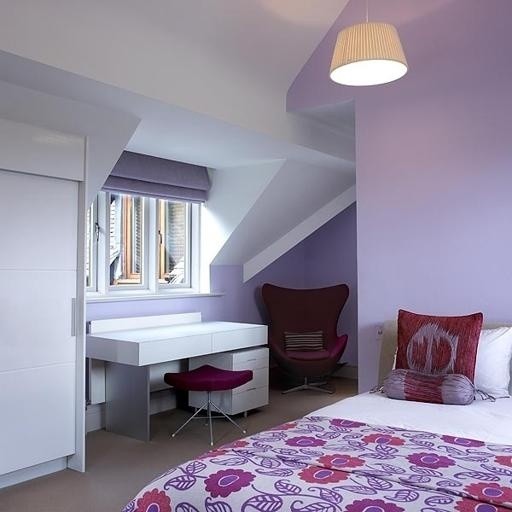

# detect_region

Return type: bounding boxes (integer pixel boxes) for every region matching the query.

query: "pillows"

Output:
[392,327,512,400]
[369,371,495,406]
[394,308,482,385]
[283,329,326,351]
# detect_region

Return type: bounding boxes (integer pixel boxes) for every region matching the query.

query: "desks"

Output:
[87,311,269,441]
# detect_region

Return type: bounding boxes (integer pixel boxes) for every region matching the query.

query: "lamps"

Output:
[330,0,408,86]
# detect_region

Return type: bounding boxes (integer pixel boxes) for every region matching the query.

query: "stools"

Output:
[163,364,255,445]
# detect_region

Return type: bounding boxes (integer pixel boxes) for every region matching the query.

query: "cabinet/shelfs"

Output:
[187,347,268,418]
[0,133,86,489]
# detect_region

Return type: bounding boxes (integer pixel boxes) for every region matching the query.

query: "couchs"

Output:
[259,283,351,395]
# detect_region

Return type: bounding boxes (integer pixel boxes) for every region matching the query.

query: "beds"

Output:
[120,387,512,512]
